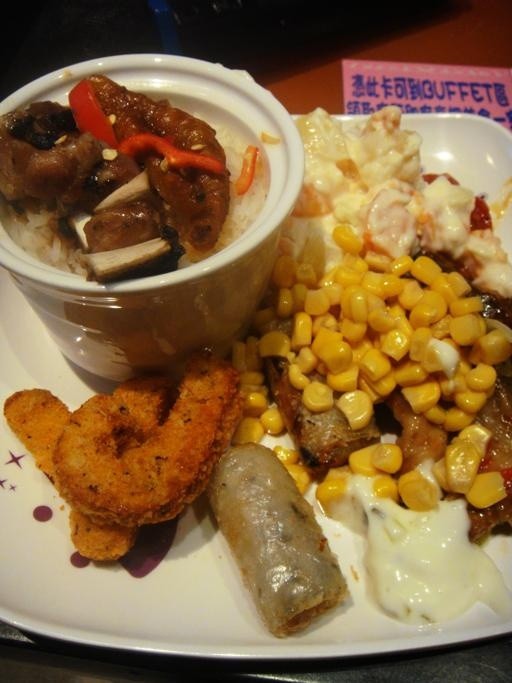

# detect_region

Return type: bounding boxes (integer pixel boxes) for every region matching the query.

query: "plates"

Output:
[2,106,510,667]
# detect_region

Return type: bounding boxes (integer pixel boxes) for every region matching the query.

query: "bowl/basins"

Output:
[0,54,305,384]
